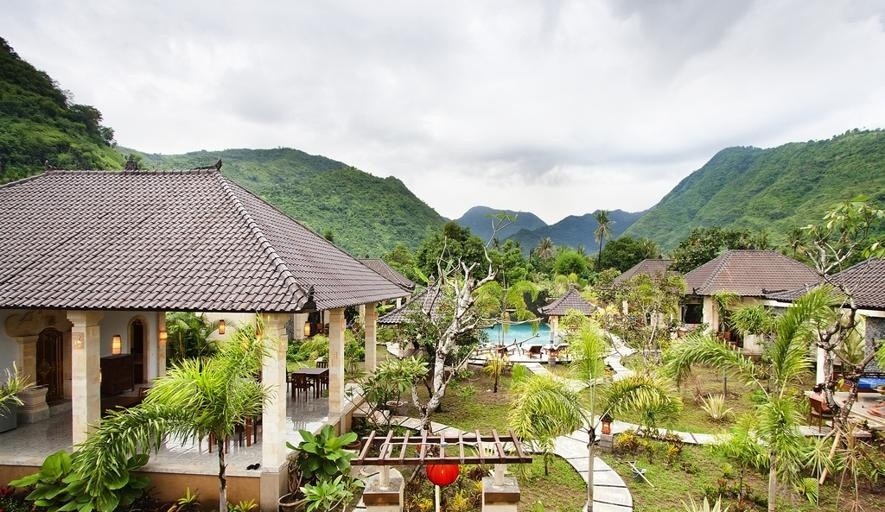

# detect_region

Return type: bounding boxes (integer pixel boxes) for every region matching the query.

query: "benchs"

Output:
[850,371,884,402]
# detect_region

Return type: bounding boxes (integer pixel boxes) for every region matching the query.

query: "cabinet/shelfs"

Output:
[100,355,133,394]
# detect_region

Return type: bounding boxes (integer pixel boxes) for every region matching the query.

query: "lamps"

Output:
[218,313,224,335]
[112,334,120,356]
[303,313,310,337]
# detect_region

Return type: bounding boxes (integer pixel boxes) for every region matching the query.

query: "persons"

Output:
[812,372,885,417]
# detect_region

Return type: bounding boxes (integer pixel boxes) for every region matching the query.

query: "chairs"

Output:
[139,387,151,398]
[291,362,329,402]
[495,343,569,359]
[808,397,835,431]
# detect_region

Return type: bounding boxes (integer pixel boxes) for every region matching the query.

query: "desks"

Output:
[102,398,141,414]
